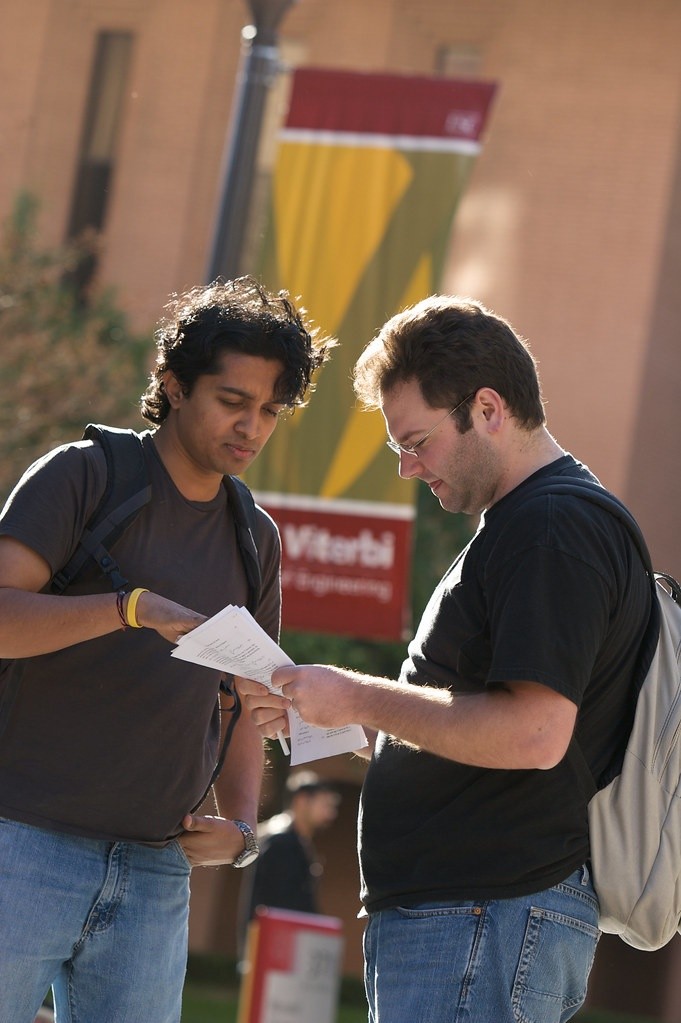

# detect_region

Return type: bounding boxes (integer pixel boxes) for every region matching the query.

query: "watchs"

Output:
[232,819,260,871]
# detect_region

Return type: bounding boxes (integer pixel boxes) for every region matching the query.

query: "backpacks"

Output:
[499,476,681,952]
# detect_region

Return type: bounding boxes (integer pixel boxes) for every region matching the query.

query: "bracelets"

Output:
[112,583,151,629]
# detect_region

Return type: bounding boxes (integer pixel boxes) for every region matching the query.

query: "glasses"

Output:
[386,393,475,459]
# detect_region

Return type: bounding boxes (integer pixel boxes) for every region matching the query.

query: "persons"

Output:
[0,270,335,1023]
[229,292,652,1023]
[237,770,344,982]
